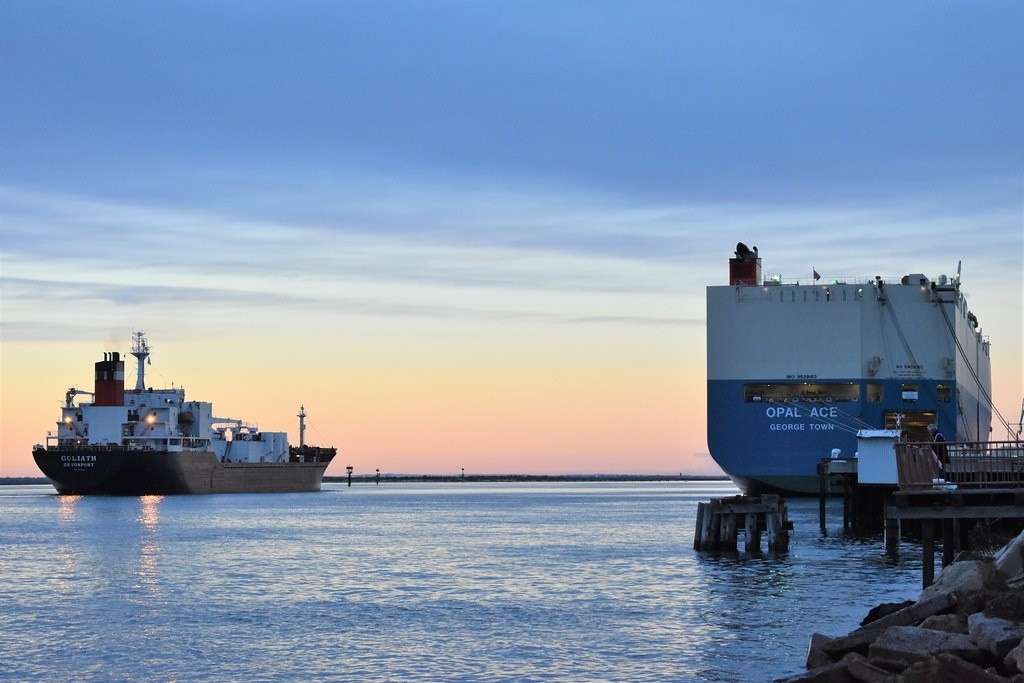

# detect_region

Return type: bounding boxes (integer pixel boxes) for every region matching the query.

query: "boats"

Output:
[705,240,993,494]
[30,331,338,493]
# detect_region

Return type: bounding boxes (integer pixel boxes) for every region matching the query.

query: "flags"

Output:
[814,272,820,280]
[147,357,151,365]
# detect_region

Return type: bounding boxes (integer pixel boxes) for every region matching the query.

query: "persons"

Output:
[927,424,950,464]
